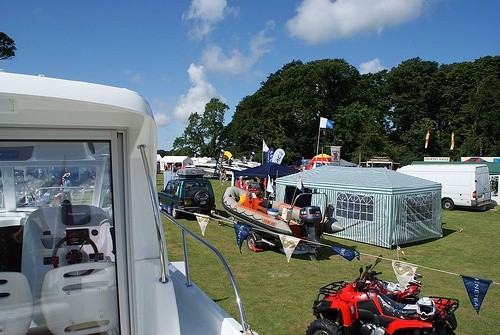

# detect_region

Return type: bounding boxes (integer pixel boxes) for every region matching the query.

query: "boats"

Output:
[222,180,344,242]
[194,161,260,174]
[0,70,259,335]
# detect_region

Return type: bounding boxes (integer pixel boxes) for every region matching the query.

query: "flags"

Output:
[320,117,334,128]
[267,147,285,165]
[215,142,225,174]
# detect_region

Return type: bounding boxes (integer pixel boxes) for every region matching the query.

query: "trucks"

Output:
[397,164,491,210]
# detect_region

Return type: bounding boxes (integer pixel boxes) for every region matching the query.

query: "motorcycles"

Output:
[307,255,460,335]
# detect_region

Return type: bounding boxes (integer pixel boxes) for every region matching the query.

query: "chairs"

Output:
[41,262,118,335]
[0,272,34,335]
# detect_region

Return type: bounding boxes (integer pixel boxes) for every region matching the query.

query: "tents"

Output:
[234,162,300,198]
[276,165,443,248]
[160,156,193,171]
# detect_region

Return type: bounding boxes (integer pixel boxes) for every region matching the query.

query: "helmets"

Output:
[416,297,435,321]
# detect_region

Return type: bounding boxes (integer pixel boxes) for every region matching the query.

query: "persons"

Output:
[238,176,244,189]
[63,176,71,200]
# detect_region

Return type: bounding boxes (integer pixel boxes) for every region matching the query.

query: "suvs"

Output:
[158,178,215,219]
